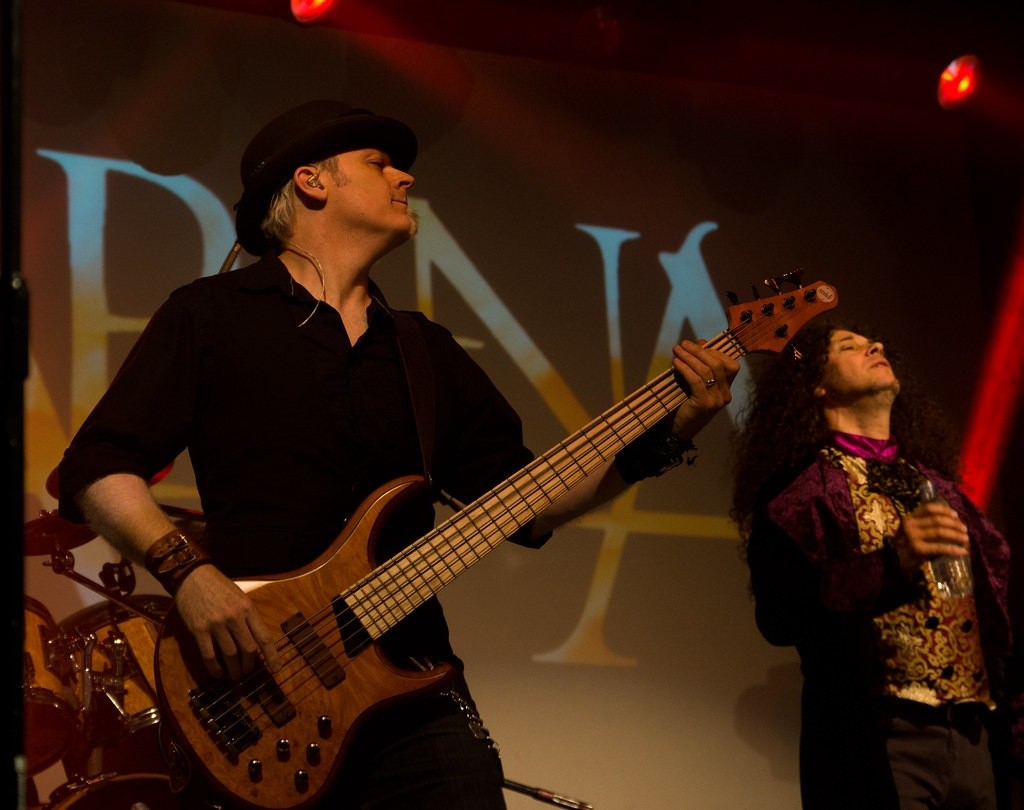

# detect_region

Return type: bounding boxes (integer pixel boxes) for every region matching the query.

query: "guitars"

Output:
[154,269,839,810]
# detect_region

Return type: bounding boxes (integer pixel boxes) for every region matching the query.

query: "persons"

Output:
[728,317,1014,810]
[56,100,741,810]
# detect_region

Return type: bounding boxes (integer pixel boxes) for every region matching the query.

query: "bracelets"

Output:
[614,422,699,486]
[144,528,212,597]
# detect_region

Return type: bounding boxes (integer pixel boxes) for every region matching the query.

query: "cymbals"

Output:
[24,510,98,556]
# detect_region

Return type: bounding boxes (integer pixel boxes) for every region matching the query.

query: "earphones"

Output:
[307,175,325,191]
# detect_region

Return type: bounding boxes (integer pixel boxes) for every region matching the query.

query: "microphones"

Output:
[118,557,136,593]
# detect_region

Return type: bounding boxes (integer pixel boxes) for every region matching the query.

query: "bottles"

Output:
[917,479,975,599]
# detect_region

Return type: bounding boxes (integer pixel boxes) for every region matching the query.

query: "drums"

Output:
[23,593,82,777]
[40,594,219,810]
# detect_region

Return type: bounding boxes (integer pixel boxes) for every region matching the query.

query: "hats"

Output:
[236,98,419,257]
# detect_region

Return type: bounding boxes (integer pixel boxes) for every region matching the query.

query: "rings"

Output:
[706,379,717,388]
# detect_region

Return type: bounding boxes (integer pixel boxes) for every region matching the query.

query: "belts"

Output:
[877,695,983,724]
